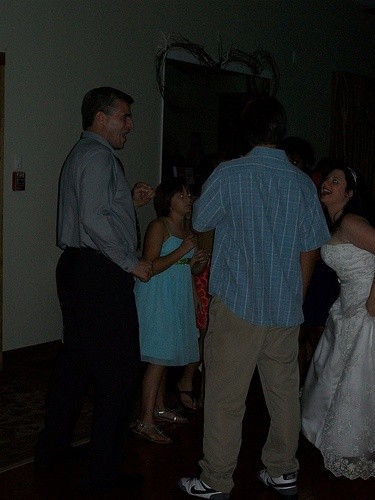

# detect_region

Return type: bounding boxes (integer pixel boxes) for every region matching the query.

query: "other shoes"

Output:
[79,468,146,491]
[34,449,88,470]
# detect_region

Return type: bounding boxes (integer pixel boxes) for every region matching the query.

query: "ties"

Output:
[115,155,141,252]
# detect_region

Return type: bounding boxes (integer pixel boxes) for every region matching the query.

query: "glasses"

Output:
[290,160,300,166]
[173,193,191,200]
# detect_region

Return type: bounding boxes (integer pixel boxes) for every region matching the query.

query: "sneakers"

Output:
[179,477,231,500]
[257,468,298,496]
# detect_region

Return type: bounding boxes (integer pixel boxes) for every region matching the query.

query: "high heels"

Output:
[132,420,173,444]
[177,382,198,411]
[153,405,189,426]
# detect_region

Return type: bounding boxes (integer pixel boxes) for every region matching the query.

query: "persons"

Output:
[131,91,375,500]
[31,85,156,500]
